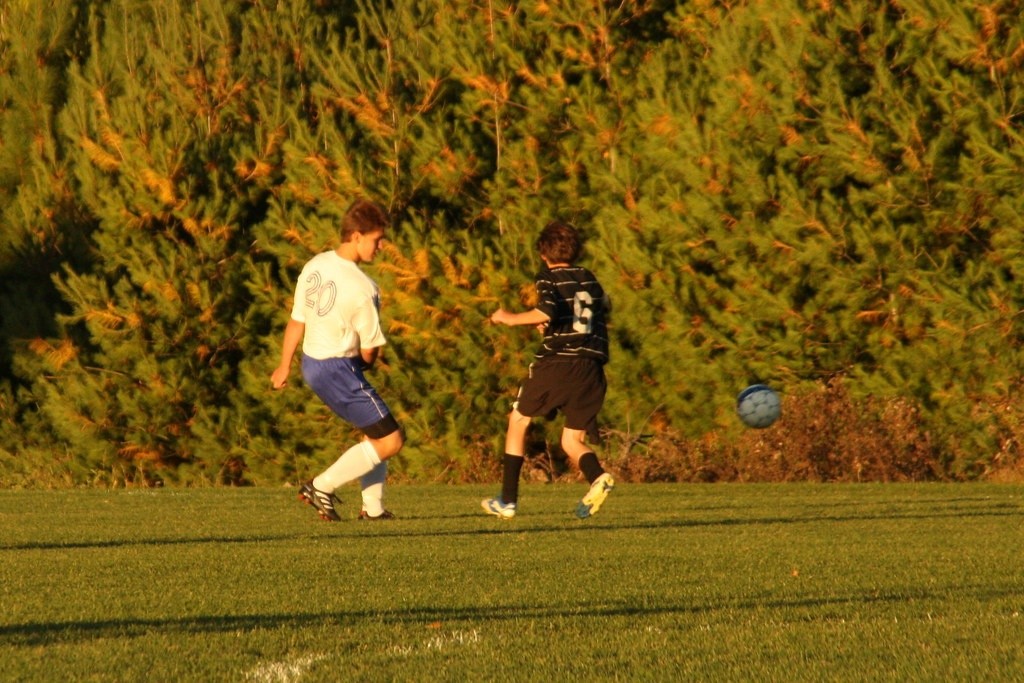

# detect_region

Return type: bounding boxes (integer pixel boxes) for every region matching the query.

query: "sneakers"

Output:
[299,478,343,521]
[577,473,614,519]
[356,509,397,520]
[481,497,517,519]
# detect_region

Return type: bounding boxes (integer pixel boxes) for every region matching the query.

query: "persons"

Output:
[480,220,614,520]
[271,193,405,521]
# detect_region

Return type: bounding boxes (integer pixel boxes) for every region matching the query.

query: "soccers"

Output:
[736,382,782,429]
